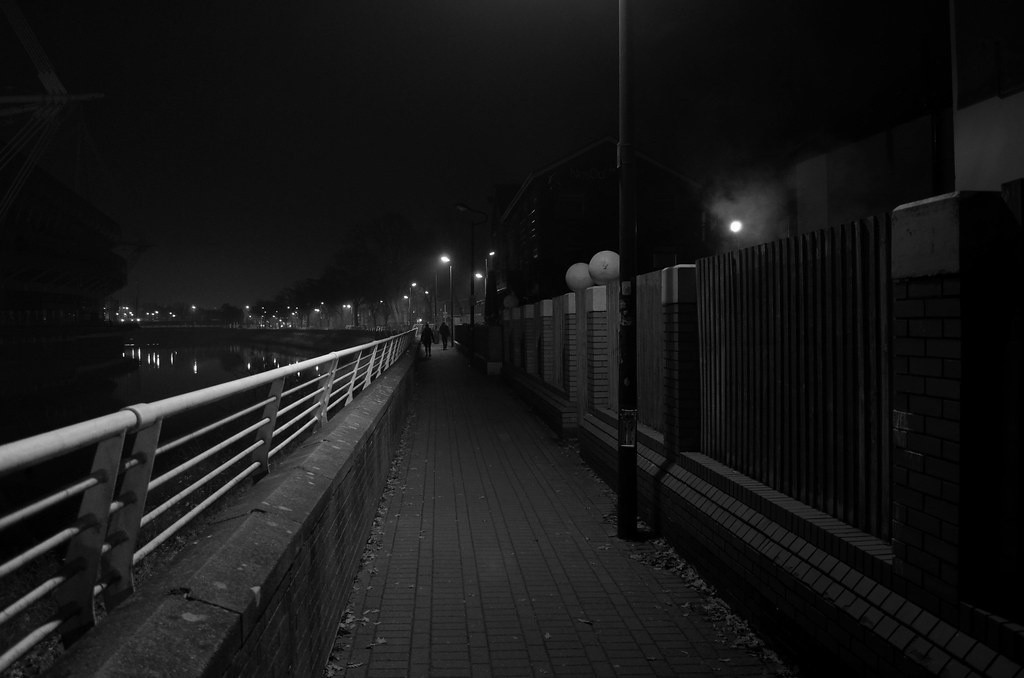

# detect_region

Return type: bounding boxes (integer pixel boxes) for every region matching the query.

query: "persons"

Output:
[434,326,439,340]
[420,323,435,359]
[439,322,450,349]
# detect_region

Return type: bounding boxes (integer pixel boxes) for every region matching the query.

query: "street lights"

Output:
[113,302,176,324]
[241,353,322,379]
[425,290,437,343]
[475,271,487,360]
[342,303,354,326]
[241,299,328,331]
[484,250,496,360]
[119,343,199,375]
[372,300,383,330]
[408,281,417,325]
[192,305,196,327]
[404,295,411,326]
[722,215,743,468]
[441,256,454,347]
